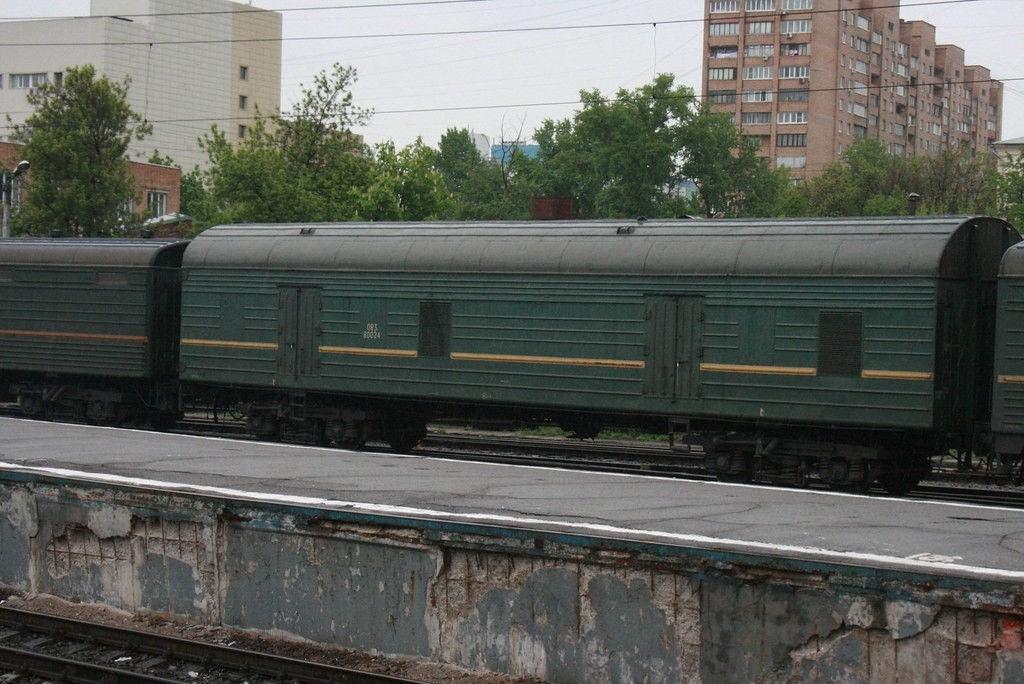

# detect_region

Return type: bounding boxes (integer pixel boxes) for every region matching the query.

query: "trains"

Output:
[0,216,1024,500]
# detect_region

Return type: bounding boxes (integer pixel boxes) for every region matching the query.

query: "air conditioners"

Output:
[900,55,903,58]
[787,34,792,38]
[763,56,768,59]
[892,51,896,56]
[799,78,805,83]
[850,70,854,74]
